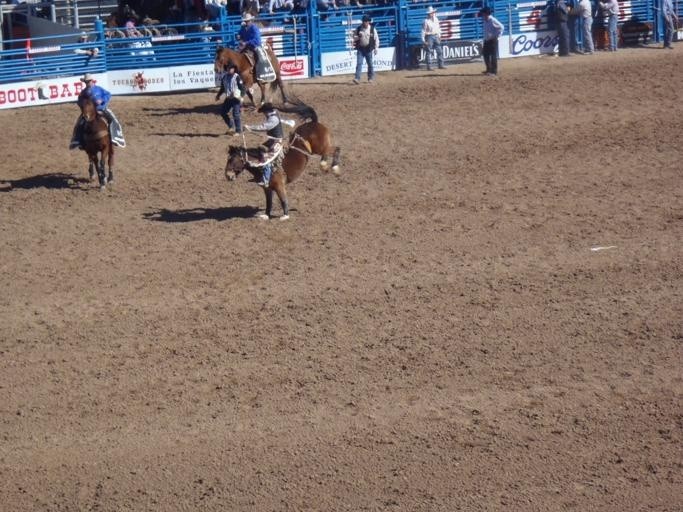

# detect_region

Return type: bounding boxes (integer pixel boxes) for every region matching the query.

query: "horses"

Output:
[212,43,287,107]
[223,90,342,222]
[79,91,116,192]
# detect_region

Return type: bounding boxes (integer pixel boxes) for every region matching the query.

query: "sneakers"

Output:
[352,79,375,84]
[228,128,234,132]
[429,66,448,71]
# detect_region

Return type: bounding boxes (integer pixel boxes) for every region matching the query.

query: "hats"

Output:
[242,13,255,22]
[257,103,272,113]
[425,6,437,14]
[80,73,97,83]
[224,62,237,72]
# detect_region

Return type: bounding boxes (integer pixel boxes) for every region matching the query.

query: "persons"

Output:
[660,1,679,50]
[568,0,595,56]
[350,14,380,85]
[422,7,446,70]
[551,0,577,57]
[596,0,621,52]
[234,12,270,86]
[240,95,284,189]
[228,0,305,24]
[317,0,483,18]
[104,2,221,61]
[73,31,98,57]
[212,60,246,137]
[475,5,504,76]
[66,72,123,149]
